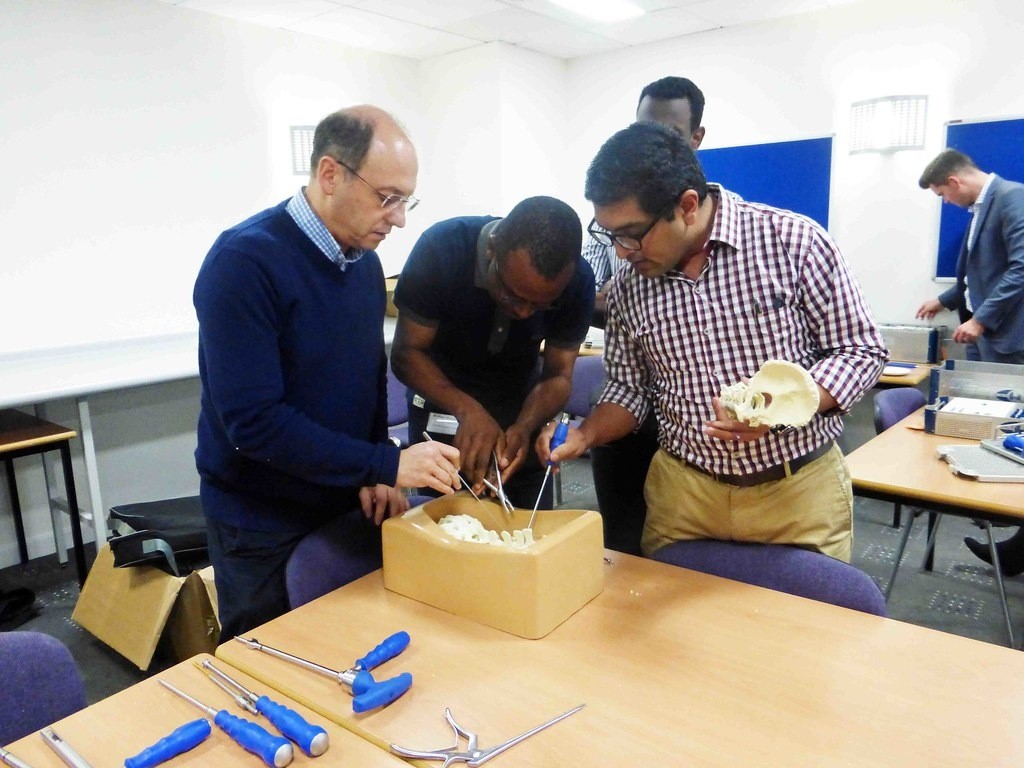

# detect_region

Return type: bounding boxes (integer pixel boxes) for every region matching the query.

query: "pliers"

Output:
[475,449,515,514]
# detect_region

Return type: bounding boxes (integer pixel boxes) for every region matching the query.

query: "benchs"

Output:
[0,408,86,592]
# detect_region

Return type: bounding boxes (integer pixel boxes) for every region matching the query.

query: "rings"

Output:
[735,432,740,440]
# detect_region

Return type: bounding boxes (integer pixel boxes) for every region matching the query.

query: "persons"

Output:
[916,146,1024,364]
[192,103,462,648]
[533,120,890,561]
[963,516,1024,577]
[389,194,597,511]
[581,74,706,327]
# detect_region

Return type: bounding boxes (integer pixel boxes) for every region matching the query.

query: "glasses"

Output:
[588,186,694,251]
[334,160,420,213]
[493,258,562,313]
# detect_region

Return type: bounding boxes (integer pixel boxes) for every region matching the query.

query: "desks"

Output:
[843,402,1024,649]
[0,546,1024,768]
[869,360,942,529]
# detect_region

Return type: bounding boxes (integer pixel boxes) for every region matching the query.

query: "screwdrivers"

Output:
[202,659,329,758]
[390,702,588,768]
[191,660,259,716]
[123,716,212,768]
[156,677,295,768]
[527,412,572,529]
[234,630,413,713]
[886,362,933,368]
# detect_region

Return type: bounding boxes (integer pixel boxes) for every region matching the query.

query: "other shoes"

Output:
[964,535,1024,576]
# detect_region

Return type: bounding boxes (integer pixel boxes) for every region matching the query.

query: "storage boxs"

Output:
[934,396,1024,441]
[386,272,401,318]
[933,359,1024,404]
[71,543,222,671]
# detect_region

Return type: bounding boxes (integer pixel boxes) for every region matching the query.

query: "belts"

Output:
[674,439,834,487]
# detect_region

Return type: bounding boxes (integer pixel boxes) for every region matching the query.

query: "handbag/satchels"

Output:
[105,495,208,575]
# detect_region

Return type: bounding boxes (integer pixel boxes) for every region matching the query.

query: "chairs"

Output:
[286,496,436,613]
[0,631,87,751]
[651,539,886,617]
[383,358,411,446]
[872,387,939,573]
[553,354,607,505]
